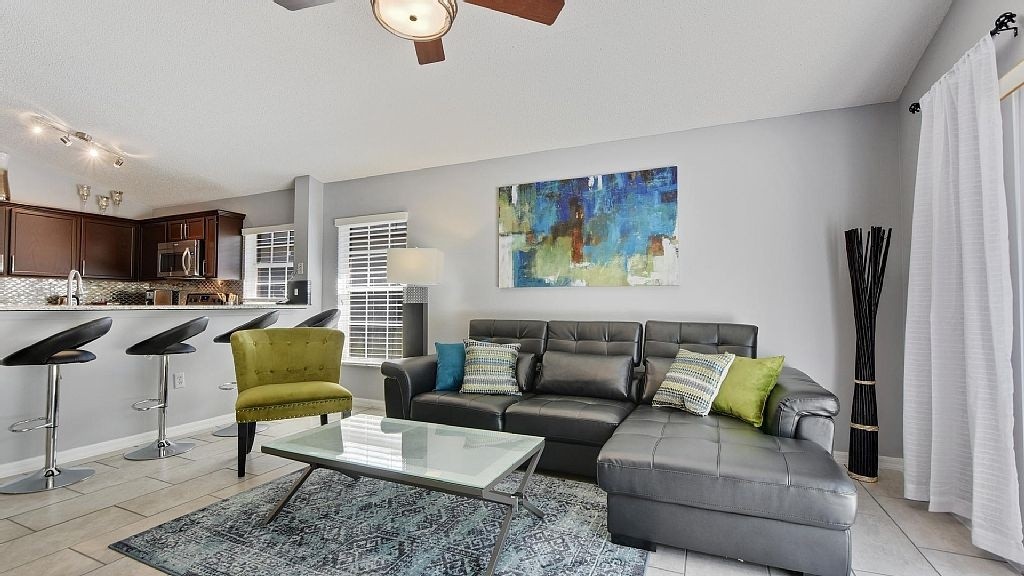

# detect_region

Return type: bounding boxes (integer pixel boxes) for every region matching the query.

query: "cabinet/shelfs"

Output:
[0,200,246,279]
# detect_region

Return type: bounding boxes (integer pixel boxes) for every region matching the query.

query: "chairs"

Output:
[229,328,354,477]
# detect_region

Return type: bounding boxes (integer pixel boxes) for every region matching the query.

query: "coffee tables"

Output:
[261,414,547,576]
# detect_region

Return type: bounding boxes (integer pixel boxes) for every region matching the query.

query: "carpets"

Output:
[109,466,648,576]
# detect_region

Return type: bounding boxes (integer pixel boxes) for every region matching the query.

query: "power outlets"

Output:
[297,263,304,275]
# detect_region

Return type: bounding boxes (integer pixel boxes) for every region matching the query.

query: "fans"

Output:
[275,0,565,64]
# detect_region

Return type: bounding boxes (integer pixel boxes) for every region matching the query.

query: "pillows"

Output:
[515,352,538,390]
[656,349,736,416]
[710,351,786,428]
[537,351,633,401]
[433,342,465,390]
[647,356,673,400]
[459,339,523,397]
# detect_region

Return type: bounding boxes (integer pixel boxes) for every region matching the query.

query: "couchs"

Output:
[381,318,858,576]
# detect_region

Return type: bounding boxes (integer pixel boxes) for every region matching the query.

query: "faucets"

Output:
[67,269,84,306]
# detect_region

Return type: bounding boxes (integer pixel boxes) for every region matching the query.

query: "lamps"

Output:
[63,132,124,209]
[371,0,459,42]
[387,247,438,285]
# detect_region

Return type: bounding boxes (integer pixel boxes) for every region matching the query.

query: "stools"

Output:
[0,318,113,494]
[211,310,279,437]
[296,309,340,327]
[124,317,206,460]
